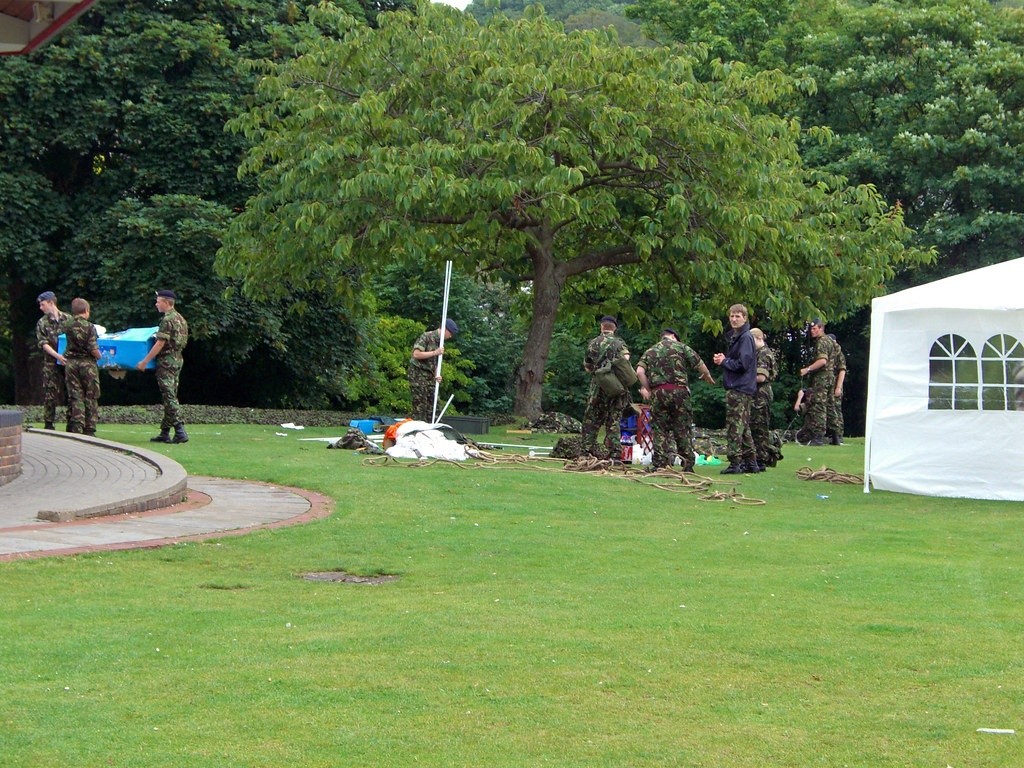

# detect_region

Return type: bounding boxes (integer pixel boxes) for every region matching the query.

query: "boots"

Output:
[808,435,823,445]
[66,426,74,432]
[150,427,170,442]
[829,435,840,445]
[166,424,188,443]
[44,422,55,430]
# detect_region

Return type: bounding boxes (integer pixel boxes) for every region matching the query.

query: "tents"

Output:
[863,255,1024,502]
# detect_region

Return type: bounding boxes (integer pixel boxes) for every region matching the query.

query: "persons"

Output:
[409,319,459,424]
[138,289,189,444]
[826,332,847,444]
[800,318,841,446]
[578,315,650,459]
[35,290,74,432]
[793,387,813,443]
[713,303,758,475]
[63,297,102,437]
[636,328,715,473]
[749,328,777,472]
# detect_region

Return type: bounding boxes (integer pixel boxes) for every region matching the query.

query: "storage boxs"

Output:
[350,420,381,434]
[57,335,157,368]
[442,415,490,435]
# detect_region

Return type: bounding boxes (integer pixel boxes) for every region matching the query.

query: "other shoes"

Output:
[810,319,820,327]
[681,467,696,475]
[742,464,759,473]
[759,463,765,471]
[828,334,837,340]
[720,463,740,474]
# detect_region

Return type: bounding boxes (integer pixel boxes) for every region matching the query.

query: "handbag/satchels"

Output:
[595,358,637,397]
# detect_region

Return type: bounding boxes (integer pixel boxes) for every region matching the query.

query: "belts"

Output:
[653,383,687,390]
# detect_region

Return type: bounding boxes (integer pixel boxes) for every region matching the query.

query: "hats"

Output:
[601,315,618,328]
[156,290,177,299]
[665,329,680,342]
[36,291,55,302]
[446,319,458,334]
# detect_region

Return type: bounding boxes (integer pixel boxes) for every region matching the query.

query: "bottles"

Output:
[620,436,632,464]
